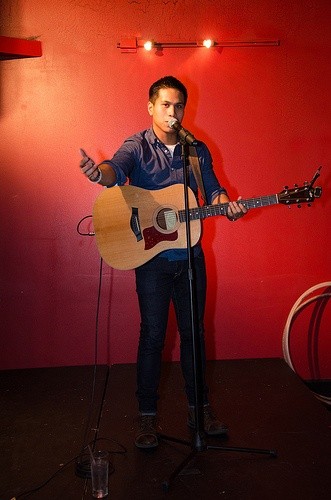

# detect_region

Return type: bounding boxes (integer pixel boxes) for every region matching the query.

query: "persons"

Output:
[79,75,248,448]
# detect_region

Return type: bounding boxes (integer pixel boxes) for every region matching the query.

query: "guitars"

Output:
[92,180,322,271]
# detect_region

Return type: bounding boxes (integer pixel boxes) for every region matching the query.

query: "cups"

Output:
[91,450,109,498]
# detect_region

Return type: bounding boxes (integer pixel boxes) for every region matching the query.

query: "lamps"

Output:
[202,38,215,48]
[144,40,153,50]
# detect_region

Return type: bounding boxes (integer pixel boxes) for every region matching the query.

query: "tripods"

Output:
[151,142,277,488]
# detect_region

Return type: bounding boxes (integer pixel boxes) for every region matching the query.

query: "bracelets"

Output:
[88,168,101,183]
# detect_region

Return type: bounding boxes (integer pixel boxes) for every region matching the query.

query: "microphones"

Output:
[168,117,198,145]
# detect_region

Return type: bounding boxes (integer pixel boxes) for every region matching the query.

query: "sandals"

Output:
[186,404,231,435]
[132,415,158,448]
